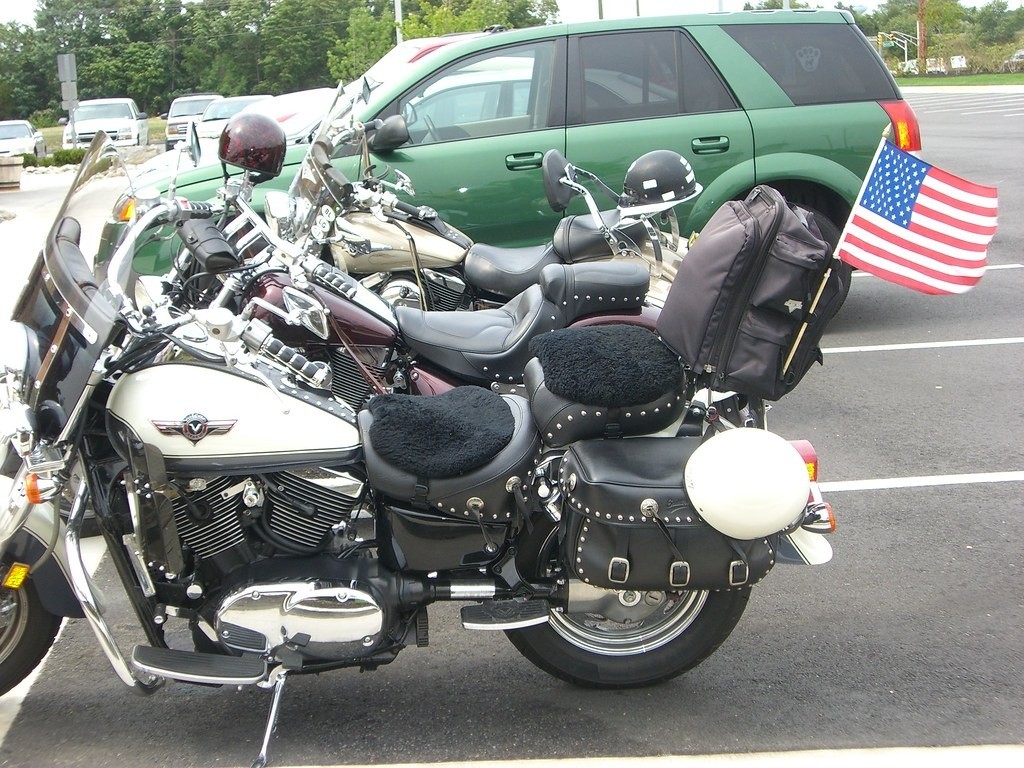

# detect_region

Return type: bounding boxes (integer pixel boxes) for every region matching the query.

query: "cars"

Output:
[0,120,47,158]
[57,97,147,154]
[240,25,677,132]
[196,93,276,142]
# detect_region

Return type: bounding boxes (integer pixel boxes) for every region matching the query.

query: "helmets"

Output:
[623,150,696,205]
[218,110,286,177]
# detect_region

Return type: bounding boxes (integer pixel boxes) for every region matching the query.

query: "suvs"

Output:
[161,93,223,151]
[92,7,923,318]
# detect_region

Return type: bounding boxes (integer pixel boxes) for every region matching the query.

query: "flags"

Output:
[833,139,1000,295]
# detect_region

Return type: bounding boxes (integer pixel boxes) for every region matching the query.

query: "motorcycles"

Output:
[0,74,836,767]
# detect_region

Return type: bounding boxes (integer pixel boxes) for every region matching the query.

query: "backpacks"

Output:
[655,184,842,400]
